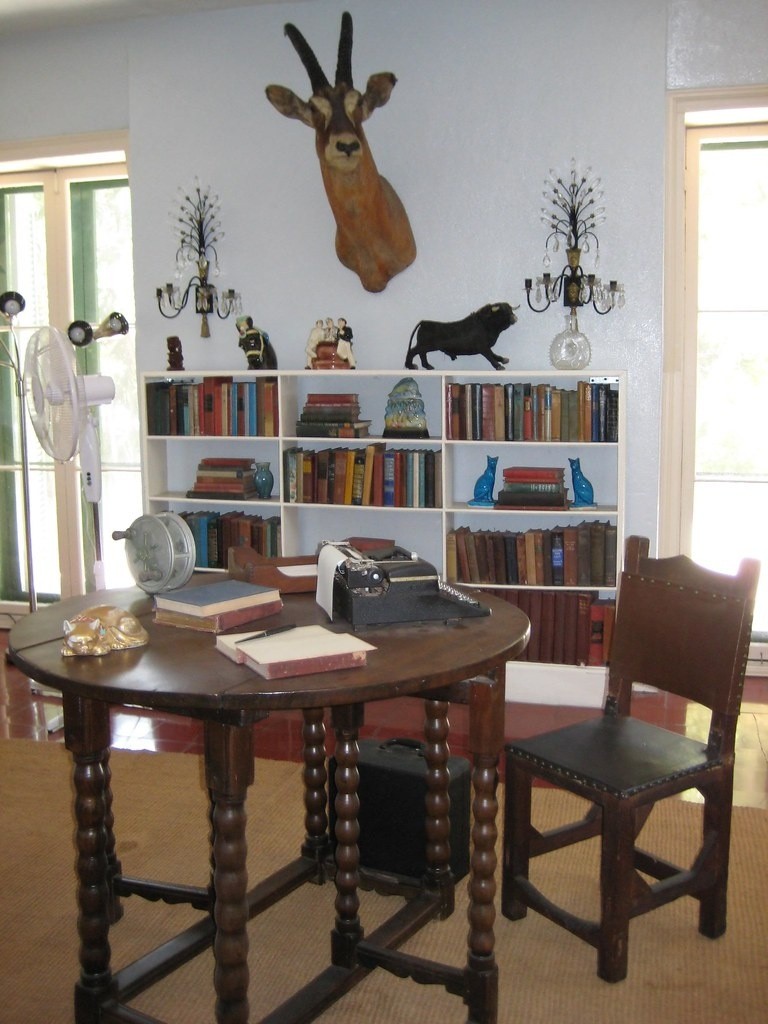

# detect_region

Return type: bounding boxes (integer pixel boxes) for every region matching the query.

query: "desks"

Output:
[7,585,531,1024]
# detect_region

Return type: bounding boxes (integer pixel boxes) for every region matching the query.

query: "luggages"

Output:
[328,737,470,884]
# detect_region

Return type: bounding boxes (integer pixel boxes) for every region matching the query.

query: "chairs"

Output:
[498,533,761,986]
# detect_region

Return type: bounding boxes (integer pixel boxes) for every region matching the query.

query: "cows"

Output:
[405,303,521,371]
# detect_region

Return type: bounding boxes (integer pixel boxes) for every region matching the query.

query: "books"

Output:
[159,509,281,570]
[479,588,616,666]
[295,393,373,438]
[445,380,618,442]
[185,457,260,501]
[146,376,279,437]
[447,520,617,586]
[153,577,378,682]
[282,441,443,508]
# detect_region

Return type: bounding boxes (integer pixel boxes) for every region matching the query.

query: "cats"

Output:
[568,457,593,506]
[474,455,499,502]
[60,604,149,657]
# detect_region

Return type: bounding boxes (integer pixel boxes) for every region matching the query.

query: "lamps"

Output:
[152,174,244,338]
[521,155,627,315]
[0,290,131,665]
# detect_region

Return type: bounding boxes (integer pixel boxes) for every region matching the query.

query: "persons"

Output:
[304,317,358,367]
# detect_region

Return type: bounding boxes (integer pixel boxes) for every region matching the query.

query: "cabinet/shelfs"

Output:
[136,369,627,709]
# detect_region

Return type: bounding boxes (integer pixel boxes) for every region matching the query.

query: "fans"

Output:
[20,325,152,734]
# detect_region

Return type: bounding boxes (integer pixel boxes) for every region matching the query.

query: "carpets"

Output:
[0,738,768,1024]
